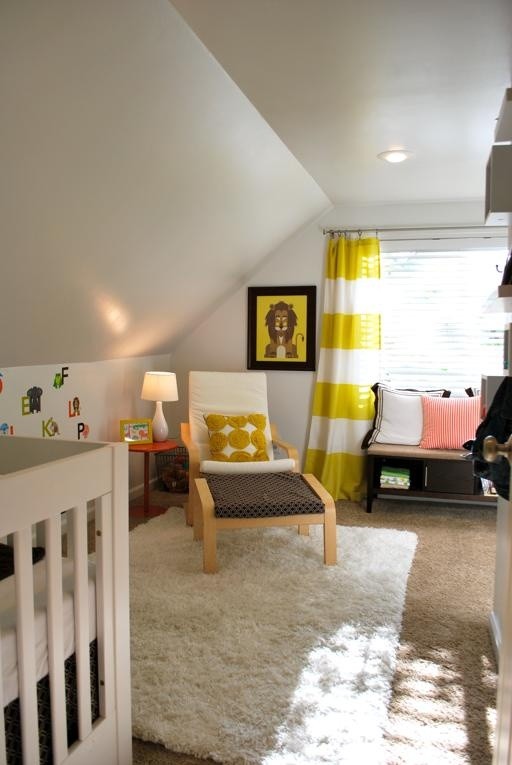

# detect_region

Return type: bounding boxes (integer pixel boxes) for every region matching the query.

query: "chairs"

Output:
[176,370,338,574]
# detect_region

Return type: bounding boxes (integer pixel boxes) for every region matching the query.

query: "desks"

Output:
[125,438,180,517]
[366,440,497,514]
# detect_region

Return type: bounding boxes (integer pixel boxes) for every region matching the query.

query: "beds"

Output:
[1,434,135,765]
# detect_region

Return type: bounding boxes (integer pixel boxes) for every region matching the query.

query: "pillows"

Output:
[201,412,269,463]
[417,394,480,451]
[360,383,452,450]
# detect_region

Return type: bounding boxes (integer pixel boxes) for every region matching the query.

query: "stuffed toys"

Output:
[159,454,189,492]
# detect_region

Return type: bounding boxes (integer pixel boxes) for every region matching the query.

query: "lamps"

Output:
[139,369,180,442]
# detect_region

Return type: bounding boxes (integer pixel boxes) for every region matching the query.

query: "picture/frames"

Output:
[247,283,319,371]
[121,418,153,444]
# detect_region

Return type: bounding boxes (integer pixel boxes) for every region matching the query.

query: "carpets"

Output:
[88,505,418,765]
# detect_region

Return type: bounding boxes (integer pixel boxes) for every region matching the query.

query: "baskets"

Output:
[154,446,190,493]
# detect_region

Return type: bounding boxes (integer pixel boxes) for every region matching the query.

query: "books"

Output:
[379,468,411,489]
